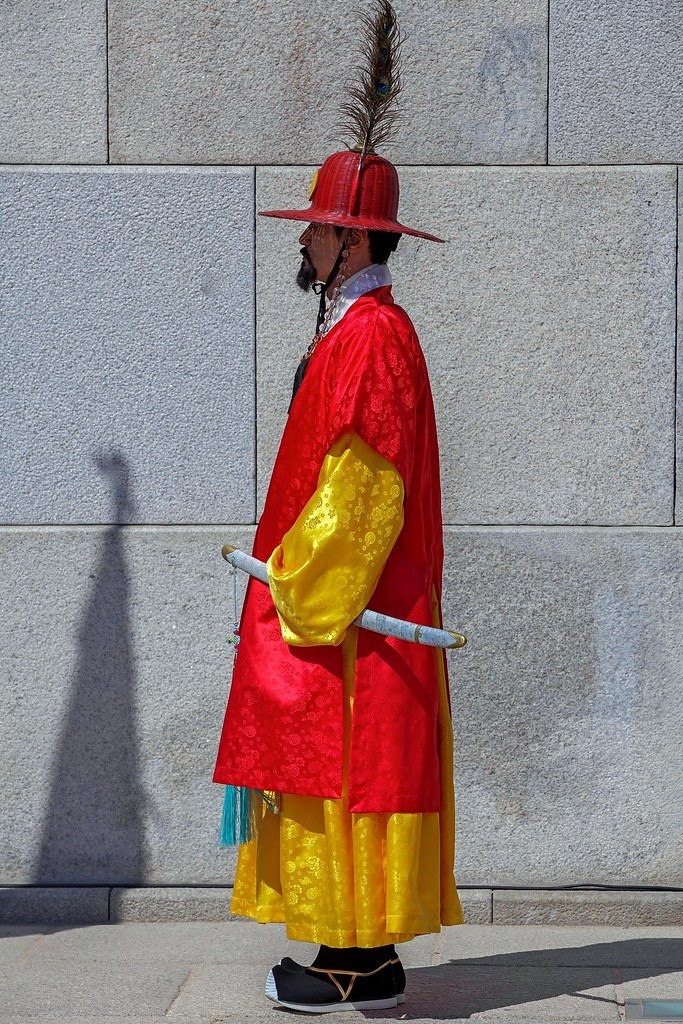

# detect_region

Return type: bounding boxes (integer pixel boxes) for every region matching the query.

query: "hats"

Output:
[258,148,446,247]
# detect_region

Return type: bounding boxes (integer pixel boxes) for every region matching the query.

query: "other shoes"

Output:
[279,945,405,1004]
[265,945,398,1013]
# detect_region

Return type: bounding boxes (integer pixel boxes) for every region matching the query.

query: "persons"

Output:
[212,146,467,1015]
